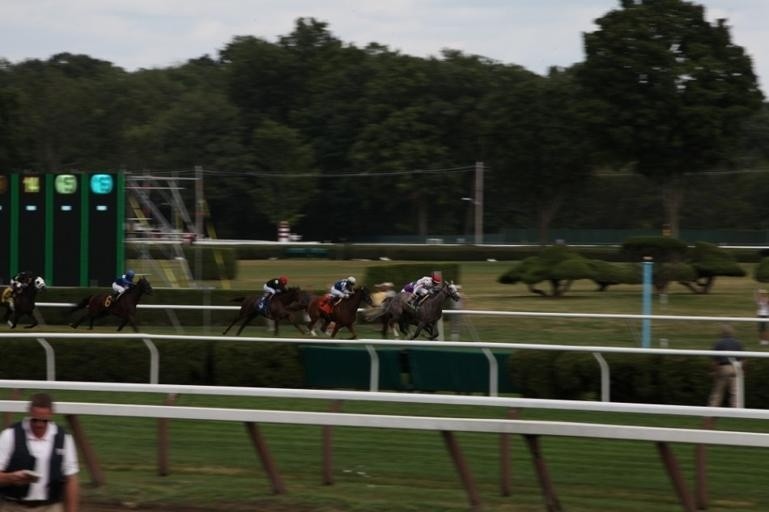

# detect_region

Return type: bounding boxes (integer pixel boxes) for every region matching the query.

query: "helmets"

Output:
[280,276,288,284]
[347,276,356,284]
[127,271,134,277]
[433,275,442,283]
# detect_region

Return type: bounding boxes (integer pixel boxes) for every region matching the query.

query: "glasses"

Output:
[30,418,48,423]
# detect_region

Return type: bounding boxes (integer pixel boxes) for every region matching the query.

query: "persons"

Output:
[755,287,769,345]
[111,268,138,304]
[2,269,31,311]
[369,282,397,308]
[706,323,746,407]
[262,276,292,296]
[329,275,358,306]
[0,393,80,511]
[400,271,443,307]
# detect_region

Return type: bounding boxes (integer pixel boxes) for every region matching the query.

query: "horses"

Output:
[222,285,308,336]
[62,275,154,333]
[361,280,462,340]
[0,272,48,329]
[286,285,373,339]
